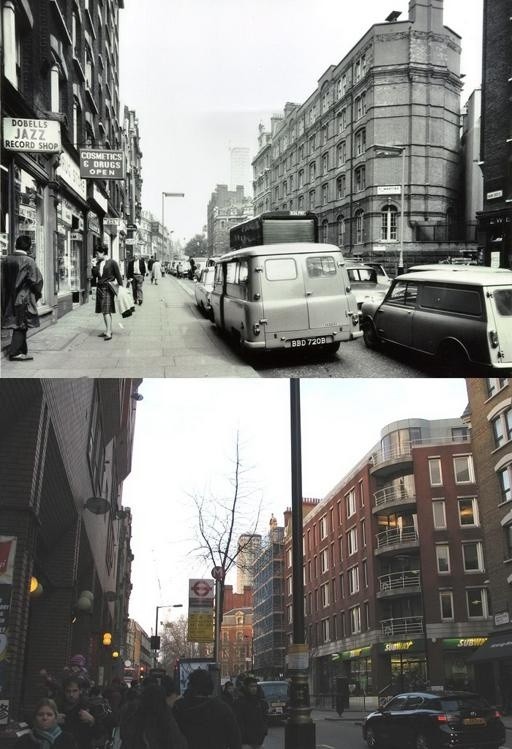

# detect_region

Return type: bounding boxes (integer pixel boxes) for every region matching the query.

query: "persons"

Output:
[140,255,203,286]
[126,253,146,306]
[1,235,44,361]
[91,244,123,341]
[17,653,270,749]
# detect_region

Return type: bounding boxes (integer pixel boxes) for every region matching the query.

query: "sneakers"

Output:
[134,299,142,305]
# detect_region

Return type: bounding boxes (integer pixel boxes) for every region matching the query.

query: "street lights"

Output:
[162,191,184,293]
[373,144,406,274]
[153,604,183,670]
[392,554,430,690]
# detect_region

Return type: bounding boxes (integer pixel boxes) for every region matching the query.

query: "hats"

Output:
[68,654,88,672]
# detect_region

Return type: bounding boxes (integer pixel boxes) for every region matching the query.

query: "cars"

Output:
[362,690,506,749]
[249,680,290,721]
[123,668,138,688]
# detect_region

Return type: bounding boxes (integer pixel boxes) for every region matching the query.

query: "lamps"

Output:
[70,591,95,624]
[103,633,112,646]
[30,575,44,598]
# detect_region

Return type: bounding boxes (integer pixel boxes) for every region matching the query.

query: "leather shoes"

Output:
[98,330,114,341]
[8,353,34,362]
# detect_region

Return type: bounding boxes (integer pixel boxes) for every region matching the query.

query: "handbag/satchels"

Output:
[109,277,120,295]
